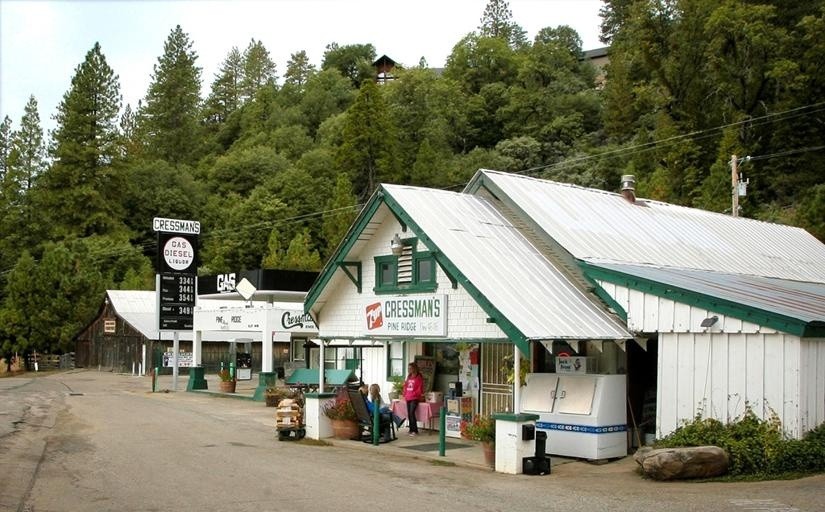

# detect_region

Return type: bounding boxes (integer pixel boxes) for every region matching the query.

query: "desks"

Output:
[391,399,444,435]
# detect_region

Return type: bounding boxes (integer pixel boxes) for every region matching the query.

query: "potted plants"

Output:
[389,375,405,403]
[264,386,284,406]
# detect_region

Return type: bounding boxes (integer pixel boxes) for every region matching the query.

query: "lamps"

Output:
[389,232,408,258]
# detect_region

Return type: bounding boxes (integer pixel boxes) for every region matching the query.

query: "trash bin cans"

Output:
[230,367,234,378]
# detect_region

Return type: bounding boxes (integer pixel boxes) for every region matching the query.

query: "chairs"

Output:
[347,389,398,445]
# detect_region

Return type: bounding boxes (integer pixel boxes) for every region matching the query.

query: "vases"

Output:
[220,382,234,393]
[480,440,495,466]
[332,417,359,440]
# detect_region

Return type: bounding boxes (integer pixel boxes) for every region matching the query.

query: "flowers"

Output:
[320,388,359,420]
[218,369,235,382]
[458,413,495,441]
[286,381,303,405]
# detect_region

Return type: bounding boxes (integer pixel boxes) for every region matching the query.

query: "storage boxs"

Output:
[424,391,444,404]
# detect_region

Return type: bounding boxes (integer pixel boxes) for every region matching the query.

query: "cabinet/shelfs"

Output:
[443,395,474,438]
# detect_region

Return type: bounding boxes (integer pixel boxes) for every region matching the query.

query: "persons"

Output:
[368,383,386,409]
[404,362,424,436]
[357,384,407,443]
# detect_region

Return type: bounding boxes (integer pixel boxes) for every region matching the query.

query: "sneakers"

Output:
[398,418,406,428]
[404,431,418,437]
